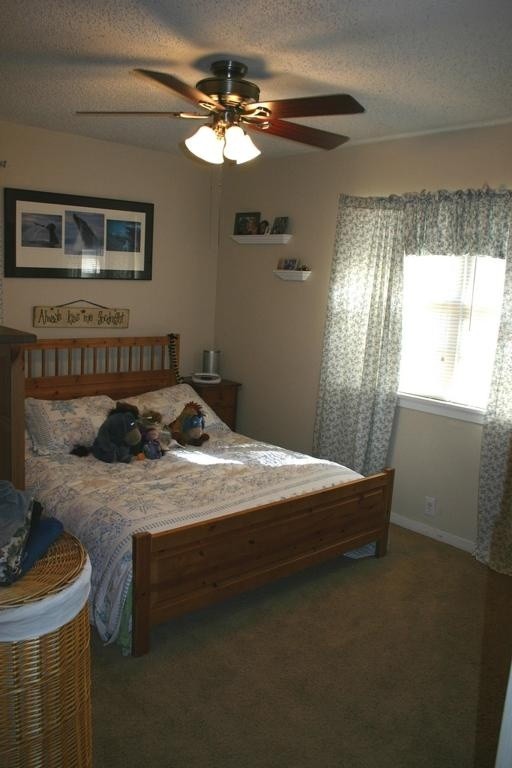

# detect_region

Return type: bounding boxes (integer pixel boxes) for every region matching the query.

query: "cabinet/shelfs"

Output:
[230,235,312,281]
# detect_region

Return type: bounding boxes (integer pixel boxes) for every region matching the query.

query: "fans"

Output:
[76,59,365,151]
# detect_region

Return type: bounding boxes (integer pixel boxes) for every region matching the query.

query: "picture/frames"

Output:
[4,187,154,280]
[234,211,261,235]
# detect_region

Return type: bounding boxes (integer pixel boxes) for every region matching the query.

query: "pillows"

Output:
[25,396,117,455]
[116,382,229,432]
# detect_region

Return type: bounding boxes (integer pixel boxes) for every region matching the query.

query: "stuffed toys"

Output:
[164,401,209,451]
[66,401,148,465]
[141,428,164,460]
[137,409,163,432]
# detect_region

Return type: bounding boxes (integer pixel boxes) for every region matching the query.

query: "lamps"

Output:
[184,111,261,165]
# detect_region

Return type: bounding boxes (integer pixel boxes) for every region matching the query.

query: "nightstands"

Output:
[182,377,241,432]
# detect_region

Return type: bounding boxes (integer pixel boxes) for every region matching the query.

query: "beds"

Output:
[22,334,395,657]
